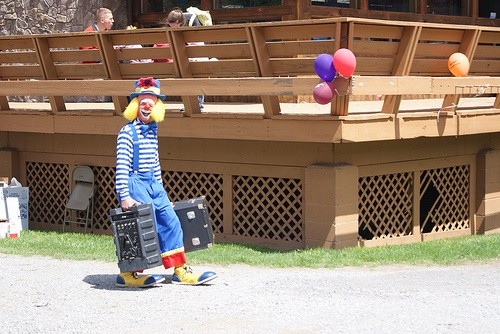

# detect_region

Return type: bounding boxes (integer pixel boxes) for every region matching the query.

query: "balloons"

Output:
[313,49,356,105]
[449,52,469,77]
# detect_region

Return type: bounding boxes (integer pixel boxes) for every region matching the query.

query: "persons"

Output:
[114,77,219,288]
[80,8,219,111]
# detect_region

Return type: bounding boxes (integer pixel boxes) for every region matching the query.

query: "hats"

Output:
[129,77,166,101]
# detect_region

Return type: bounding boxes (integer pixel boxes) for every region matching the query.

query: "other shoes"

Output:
[113,271,165,288]
[170,266,217,286]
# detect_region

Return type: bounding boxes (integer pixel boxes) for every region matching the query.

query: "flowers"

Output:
[137,77,156,89]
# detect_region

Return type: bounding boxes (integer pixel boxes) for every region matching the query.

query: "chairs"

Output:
[63,166,96,233]
